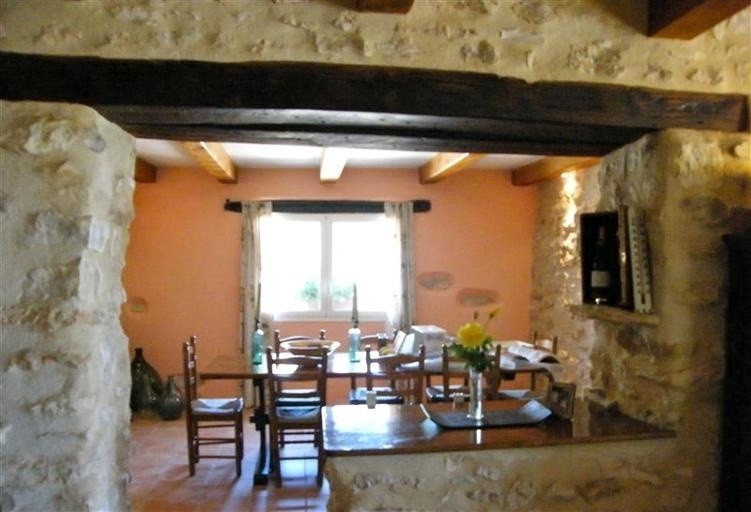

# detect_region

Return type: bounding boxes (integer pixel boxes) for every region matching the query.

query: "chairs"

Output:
[182,336,245,476]
[364,344,426,405]
[426,343,501,402]
[272,330,325,447]
[351,335,402,405]
[263,346,329,488]
[498,330,558,401]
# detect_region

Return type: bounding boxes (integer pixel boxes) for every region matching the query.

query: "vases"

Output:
[466,365,484,419]
[159,381,182,421]
[133,375,158,414]
[130,348,160,392]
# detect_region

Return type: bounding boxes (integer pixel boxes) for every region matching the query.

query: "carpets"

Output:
[162,374,185,407]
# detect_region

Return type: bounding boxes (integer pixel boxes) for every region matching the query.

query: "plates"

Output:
[420,400,553,430]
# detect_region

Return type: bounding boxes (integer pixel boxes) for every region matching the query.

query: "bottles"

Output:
[252,318,263,367]
[348,322,360,363]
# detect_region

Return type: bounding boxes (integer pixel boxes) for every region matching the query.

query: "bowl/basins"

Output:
[280,339,341,356]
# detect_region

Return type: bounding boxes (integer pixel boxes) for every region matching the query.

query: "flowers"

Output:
[447,307,499,372]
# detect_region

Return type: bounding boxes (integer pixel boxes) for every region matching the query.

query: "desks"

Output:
[320,396,675,510]
[201,351,549,485]
